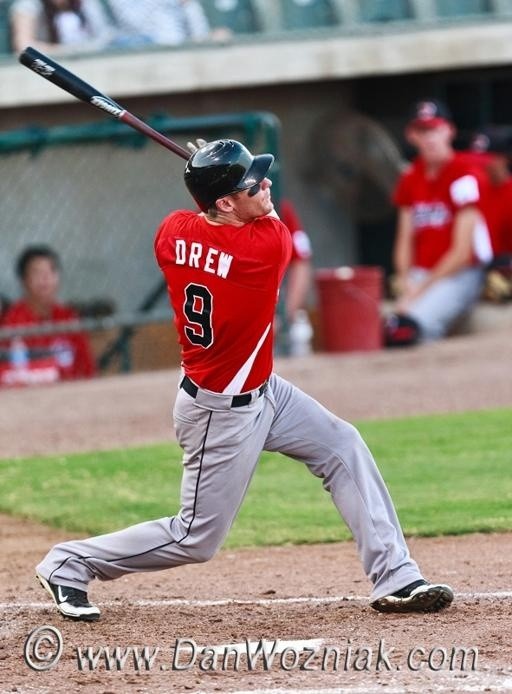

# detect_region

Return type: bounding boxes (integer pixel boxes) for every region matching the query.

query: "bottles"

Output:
[7,334,33,386]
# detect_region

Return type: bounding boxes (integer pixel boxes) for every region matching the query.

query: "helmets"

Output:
[183,138,275,214]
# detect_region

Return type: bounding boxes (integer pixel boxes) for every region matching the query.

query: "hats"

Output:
[406,99,454,130]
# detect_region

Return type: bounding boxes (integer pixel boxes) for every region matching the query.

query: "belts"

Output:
[180,374,269,408]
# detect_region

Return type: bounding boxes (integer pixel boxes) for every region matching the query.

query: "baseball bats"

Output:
[18,46,194,159]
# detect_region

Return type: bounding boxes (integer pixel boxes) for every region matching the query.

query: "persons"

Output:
[378,95,493,345]
[1,243,100,389]
[468,149,512,305]
[273,195,315,326]
[32,134,456,620]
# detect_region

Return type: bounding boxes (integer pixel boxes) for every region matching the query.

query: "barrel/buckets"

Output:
[314,266,383,352]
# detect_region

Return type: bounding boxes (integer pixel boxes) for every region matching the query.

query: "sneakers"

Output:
[372,580,456,615]
[35,575,103,621]
